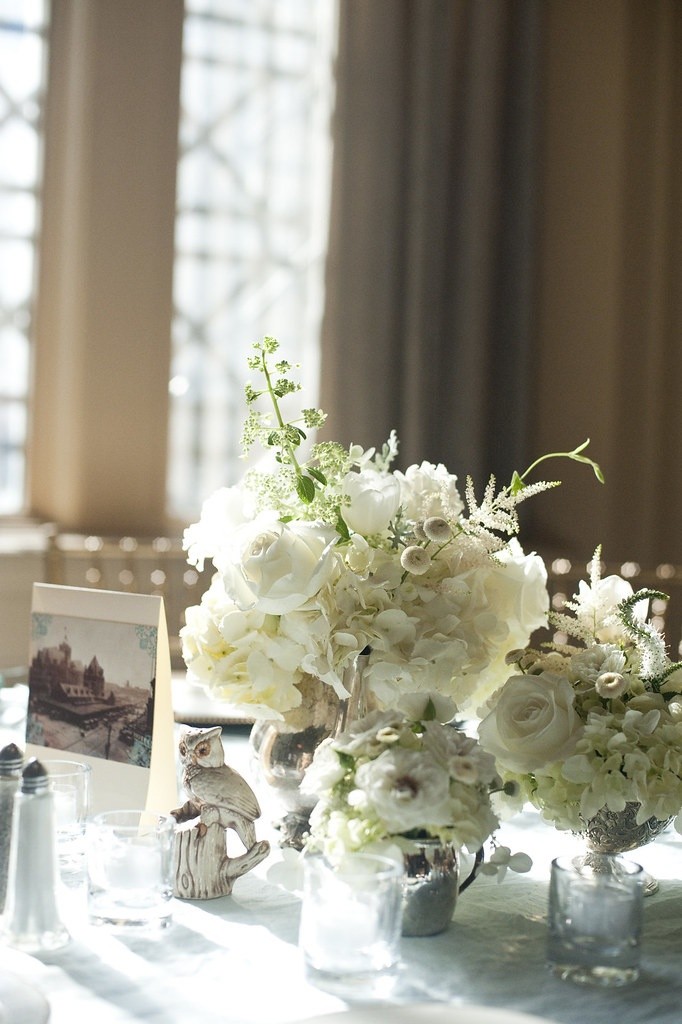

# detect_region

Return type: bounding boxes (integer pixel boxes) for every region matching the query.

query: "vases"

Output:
[543,851,660,988]
[250,646,374,786]
[380,828,484,935]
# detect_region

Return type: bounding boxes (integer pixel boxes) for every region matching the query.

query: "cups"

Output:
[90,809,174,933]
[298,852,408,975]
[34,760,93,902]
[551,853,646,988]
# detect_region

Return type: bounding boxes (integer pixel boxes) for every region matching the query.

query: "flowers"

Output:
[180,314,682,881]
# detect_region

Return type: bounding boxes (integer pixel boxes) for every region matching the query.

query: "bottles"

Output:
[0,745,22,915]
[13,761,54,930]
